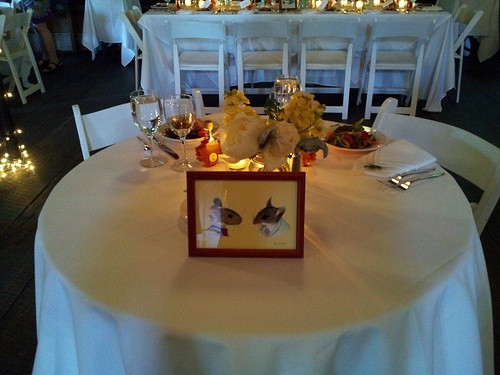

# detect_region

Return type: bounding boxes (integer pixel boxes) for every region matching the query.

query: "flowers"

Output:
[210,89,329,172]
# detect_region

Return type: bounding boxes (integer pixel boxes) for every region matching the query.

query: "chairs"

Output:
[70,6,500,238]
[0,6,47,106]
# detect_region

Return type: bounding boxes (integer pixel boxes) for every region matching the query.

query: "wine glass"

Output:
[165,0,172,12]
[163,94,203,172]
[130,89,167,168]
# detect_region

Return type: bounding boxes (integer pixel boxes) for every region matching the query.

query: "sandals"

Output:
[37,59,63,75]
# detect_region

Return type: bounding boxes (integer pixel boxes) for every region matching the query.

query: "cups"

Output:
[270,73,301,109]
[175,0,410,14]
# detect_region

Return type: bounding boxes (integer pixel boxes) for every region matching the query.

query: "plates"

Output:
[321,138,380,154]
[157,119,221,145]
[412,3,443,11]
[151,3,174,8]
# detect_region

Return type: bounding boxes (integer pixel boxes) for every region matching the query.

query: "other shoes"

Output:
[4,138,22,160]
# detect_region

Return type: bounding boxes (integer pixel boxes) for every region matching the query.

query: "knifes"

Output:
[152,135,179,160]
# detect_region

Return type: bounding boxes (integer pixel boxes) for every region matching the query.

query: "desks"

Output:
[32,115,494,375]
[141,9,455,112]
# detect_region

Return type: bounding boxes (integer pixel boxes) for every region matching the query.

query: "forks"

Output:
[375,168,446,191]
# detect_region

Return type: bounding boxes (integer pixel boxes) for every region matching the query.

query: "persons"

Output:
[14,0,63,74]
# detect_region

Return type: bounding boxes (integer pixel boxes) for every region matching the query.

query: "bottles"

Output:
[195,121,223,168]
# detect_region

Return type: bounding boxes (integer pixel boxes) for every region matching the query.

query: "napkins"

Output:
[356,139,437,178]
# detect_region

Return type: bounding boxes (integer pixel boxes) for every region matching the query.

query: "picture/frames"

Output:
[186,170,306,259]
